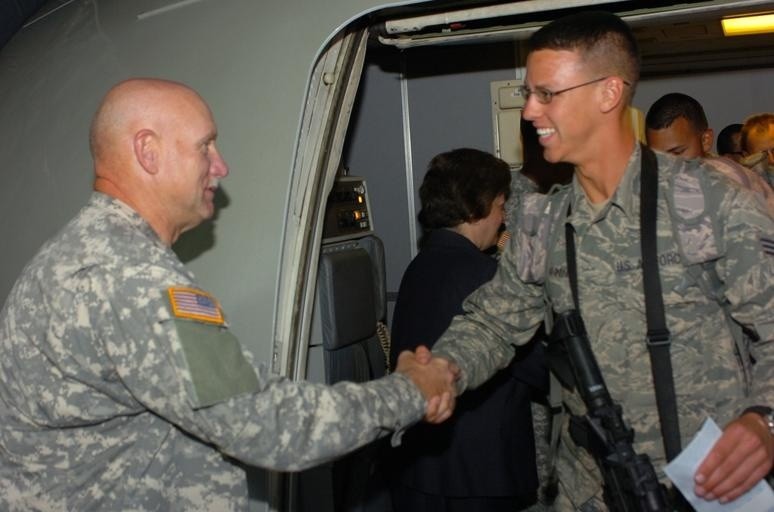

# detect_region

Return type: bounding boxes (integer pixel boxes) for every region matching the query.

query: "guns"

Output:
[539,307,675,511]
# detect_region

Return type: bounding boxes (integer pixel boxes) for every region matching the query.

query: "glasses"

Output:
[519,76,630,103]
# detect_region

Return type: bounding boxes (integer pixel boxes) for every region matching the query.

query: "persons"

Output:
[0,78,455,512]
[388,11,773,512]
